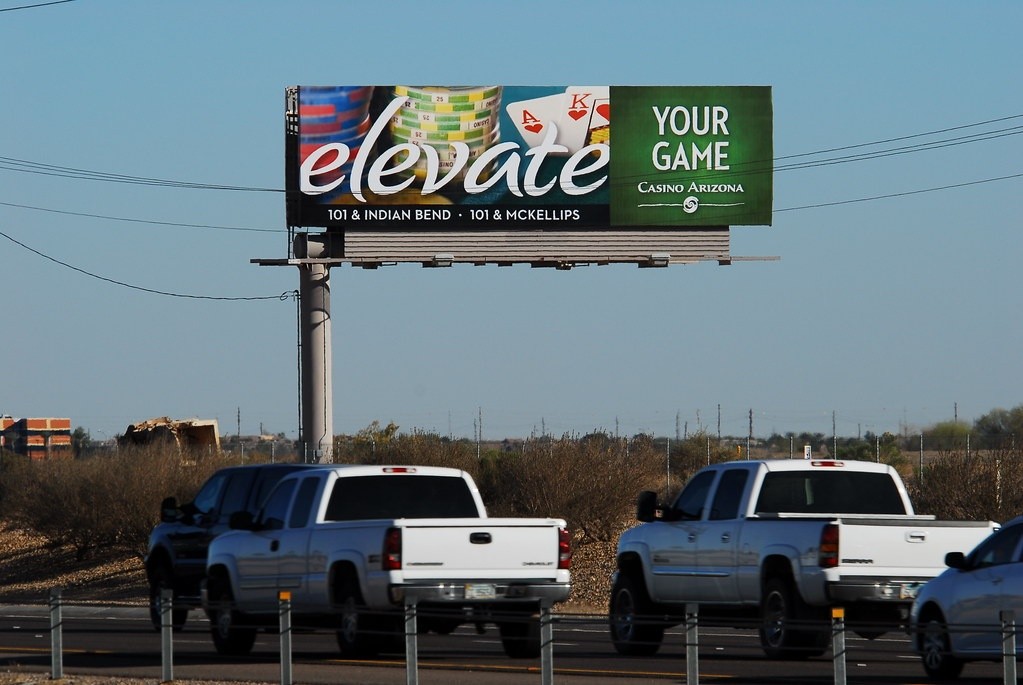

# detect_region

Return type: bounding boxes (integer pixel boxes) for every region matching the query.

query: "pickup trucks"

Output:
[608,458,1003,660]
[203,465,572,658]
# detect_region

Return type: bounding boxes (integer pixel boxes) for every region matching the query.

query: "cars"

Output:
[907,516,1023,685]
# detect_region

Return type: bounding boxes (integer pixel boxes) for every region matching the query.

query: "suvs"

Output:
[139,464,318,632]
